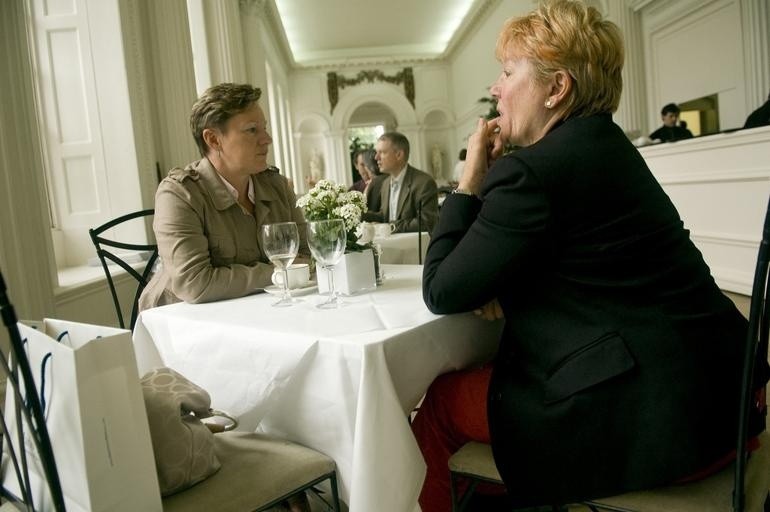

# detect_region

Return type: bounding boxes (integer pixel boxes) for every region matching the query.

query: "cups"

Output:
[270,264,311,288]
[375,222,396,238]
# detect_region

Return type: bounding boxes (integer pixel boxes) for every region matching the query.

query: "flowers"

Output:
[296,178,378,251]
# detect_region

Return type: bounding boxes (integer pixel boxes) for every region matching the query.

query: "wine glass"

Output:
[259,221,307,310]
[305,219,352,311]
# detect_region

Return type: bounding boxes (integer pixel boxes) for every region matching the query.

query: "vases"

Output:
[314,249,376,295]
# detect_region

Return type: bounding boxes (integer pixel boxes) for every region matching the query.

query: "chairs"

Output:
[89,208,159,329]
[0,269,343,512]
[443,191,770,511]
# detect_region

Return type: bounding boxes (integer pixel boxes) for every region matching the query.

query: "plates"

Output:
[263,281,318,298]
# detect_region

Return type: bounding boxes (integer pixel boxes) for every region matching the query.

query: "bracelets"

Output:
[448,189,480,199]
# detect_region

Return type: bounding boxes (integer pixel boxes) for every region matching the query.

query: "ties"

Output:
[389,181,399,222]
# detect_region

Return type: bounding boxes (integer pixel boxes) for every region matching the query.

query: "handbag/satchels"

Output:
[140,367,240,497]
[0,317,164,512]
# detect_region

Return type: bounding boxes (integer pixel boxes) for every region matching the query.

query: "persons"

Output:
[137,84,318,315]
[360,150,383,198]
[421,0,768,508]
[345,150,370,194]
[452,149,467,183]
[362,134,438,234]
[648,103,693,139]
